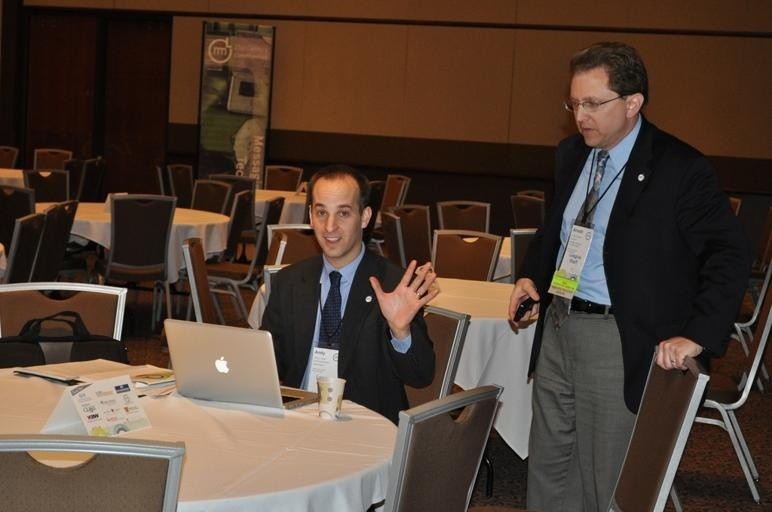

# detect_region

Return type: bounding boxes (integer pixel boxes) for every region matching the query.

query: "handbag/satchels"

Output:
[0,310,129,368]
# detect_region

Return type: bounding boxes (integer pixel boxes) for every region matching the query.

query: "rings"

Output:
[671,360,676,365]
[416,291,422,297]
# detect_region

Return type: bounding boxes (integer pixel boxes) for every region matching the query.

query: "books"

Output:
[12,357,178,401]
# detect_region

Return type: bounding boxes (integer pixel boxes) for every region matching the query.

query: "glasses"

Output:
[563,92,633,114]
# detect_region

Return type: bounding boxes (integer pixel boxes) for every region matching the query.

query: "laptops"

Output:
[165,318,320,411]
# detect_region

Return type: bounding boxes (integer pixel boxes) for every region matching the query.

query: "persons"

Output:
[504,39,749,512]
[253,162,442,426]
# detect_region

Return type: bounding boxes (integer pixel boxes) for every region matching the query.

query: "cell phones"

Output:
[513,296,538,323]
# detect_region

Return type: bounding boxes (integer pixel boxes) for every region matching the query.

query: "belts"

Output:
[571,296,617,316]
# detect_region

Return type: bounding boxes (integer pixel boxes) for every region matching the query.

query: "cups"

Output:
[317,376,346,420]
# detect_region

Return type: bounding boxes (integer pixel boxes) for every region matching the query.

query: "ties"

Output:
[319,269,346,378]
[549,150,611,332]
